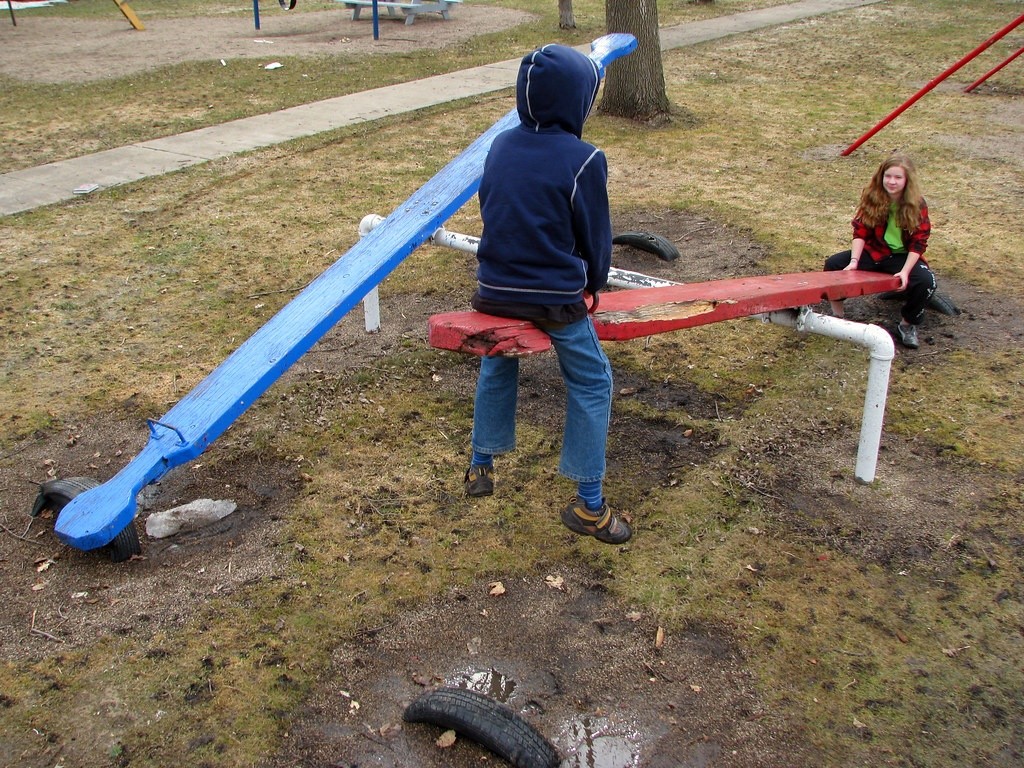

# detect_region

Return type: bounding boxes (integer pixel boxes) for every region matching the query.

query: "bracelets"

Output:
[851,258,858,261]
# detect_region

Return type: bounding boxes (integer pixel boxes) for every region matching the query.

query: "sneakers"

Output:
[463,464,495,498]
[561,493,632,544]
[896,323,920,349]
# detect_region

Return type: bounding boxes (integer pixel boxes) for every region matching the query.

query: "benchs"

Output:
[345,0,462,26]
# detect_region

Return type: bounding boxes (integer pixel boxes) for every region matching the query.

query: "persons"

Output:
[462,43,633,543]
[821,154,937,348]
